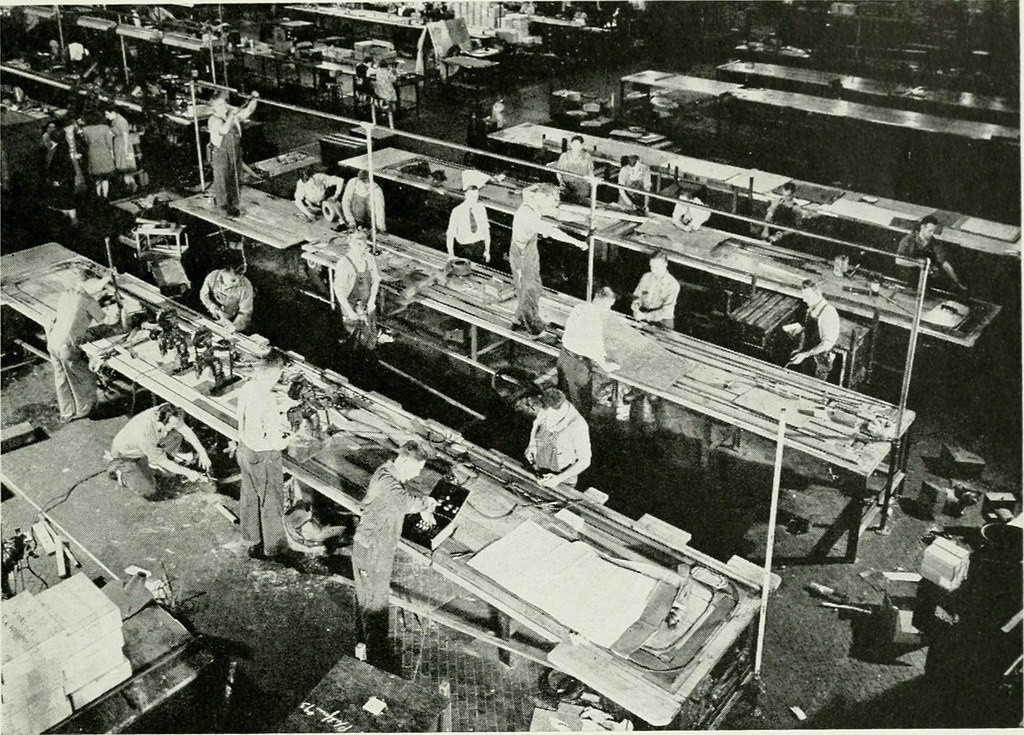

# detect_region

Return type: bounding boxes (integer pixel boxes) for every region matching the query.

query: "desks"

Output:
[0,5,1024,733]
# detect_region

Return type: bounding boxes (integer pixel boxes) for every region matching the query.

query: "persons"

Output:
[62,38,90,75]
[239,352,304,562]
[0,83,141,201]
[351,441,442,663]
[556,134,960,293]
[557,287,621,417]
[524,387,592,488]
[354,55,398,107]
[783,280,840,382]
[206,95,257,218]
[623,251,681,404]
[335,231,380,360]
[291,161,387,234]
[200,255,254,333]
[46,268,117,421]
[106,404,211,497]
[446,186,492,267]
[508,183,588,344]
[196,24,217,79]
